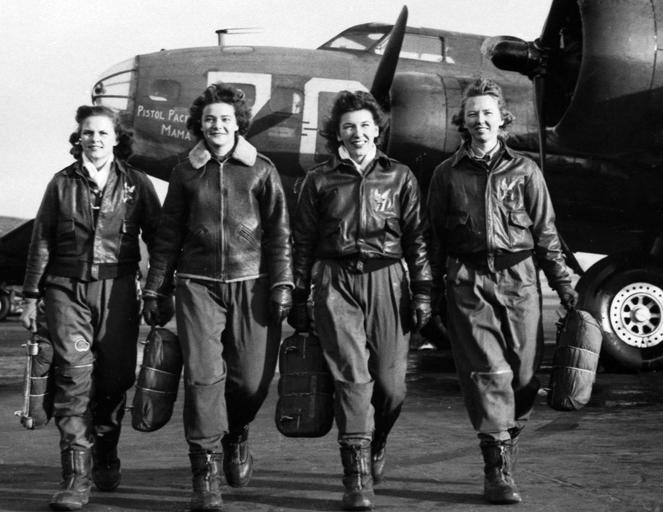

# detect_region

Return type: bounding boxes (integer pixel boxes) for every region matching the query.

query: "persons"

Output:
[17,104,173,511]
[141,81,296,512]
[423,77,580,503]
[284,90,434,509]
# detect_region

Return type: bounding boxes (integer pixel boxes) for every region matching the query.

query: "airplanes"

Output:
[0,213,39,320]
[88,0,663,374]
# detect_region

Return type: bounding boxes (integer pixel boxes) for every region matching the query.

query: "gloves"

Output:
[410,293,432,330]
[142,297,175,327]
[555,283,579,309]
[287,302,311,333]
[19,298,37,328]
[270,285,292,322]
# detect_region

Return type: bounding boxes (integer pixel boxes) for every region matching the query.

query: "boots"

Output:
[339,445,386,511]
[222,424,253,488]
[91,440,121,492]
[49,447,94,510]
[477,426,526,504]
[187,452,224,512]
